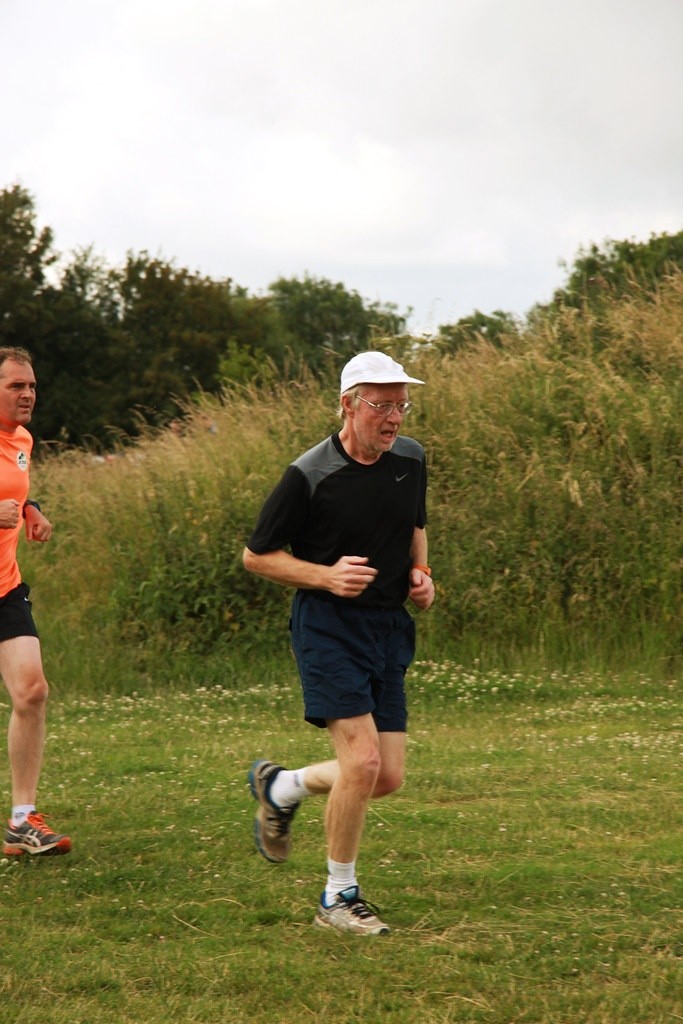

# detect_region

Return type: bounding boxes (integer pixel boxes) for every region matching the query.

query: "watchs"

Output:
[24,499,41,520]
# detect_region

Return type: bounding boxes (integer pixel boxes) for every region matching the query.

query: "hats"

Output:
[340,351,426,395]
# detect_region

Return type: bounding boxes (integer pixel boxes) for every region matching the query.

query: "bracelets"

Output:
[414,563,432,576]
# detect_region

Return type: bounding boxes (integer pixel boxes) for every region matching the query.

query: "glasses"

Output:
[355,395,416,417]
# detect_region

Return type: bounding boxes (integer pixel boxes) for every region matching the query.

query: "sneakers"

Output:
[3,809,72,858]
[247,758,301,862]
[313,886,390,938]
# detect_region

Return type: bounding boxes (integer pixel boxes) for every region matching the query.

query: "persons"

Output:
[0,347,72,855]
[240,349,434,936]
